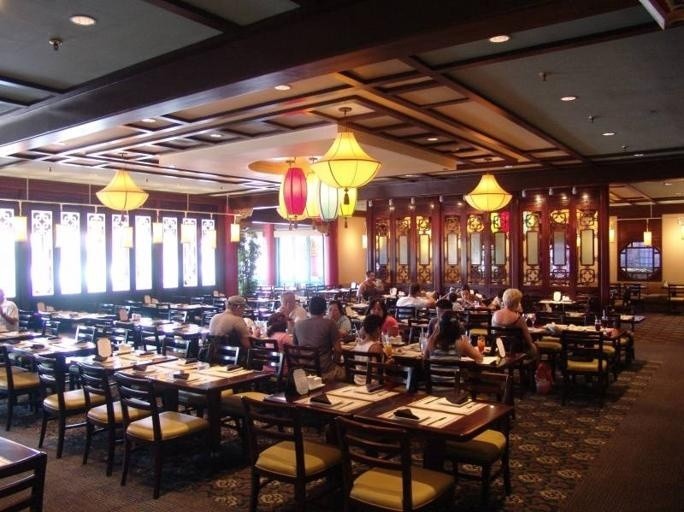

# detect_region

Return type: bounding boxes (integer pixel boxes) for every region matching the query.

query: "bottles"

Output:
[118,307,128,321]
[131,311,141,322]
[250,322,263,338]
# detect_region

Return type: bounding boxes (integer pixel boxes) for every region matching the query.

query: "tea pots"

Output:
[389,287,397,295]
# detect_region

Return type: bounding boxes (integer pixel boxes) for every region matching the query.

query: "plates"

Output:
[19,337,244,381]
[351,385,383,395]
[438,397,471,407]
[395,409,429,423]
[307,397,343,408]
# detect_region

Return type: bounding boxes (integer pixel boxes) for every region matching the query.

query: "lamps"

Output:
[463,155,512,216]
[94,151,150,221]
[274,156,358,233]
[309,106,382,205]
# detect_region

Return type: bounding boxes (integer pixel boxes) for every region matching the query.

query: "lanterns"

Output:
[283,167,308,217]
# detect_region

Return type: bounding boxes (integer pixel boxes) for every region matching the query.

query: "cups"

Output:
[522,313,528,321]
[461,335,471,344]
[477,336,486,354]
[473,299,479,309]
[420,331,427,352]
[594,318,601,333]
[382,337,392,355]
[354,329,362,343]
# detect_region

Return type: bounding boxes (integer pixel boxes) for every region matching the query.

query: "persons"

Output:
[0,289,20,363]
[356,270,541,387]
[206,294,352,383]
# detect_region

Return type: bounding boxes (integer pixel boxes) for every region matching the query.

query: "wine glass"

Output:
[601,315,609,335]
[529,312,537,331]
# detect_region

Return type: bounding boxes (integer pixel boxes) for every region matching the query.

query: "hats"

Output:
[227,295,252,309]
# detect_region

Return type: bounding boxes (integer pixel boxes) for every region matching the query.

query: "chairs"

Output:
[239,394,344,511]
[76,360,151,478]
[430,362,519,510]
[333,414,456,512]
[31,352,106,460]
[0,344,42,433]
[114,371,213,501]
[0,435,51,512]
[1,280,684,401]
